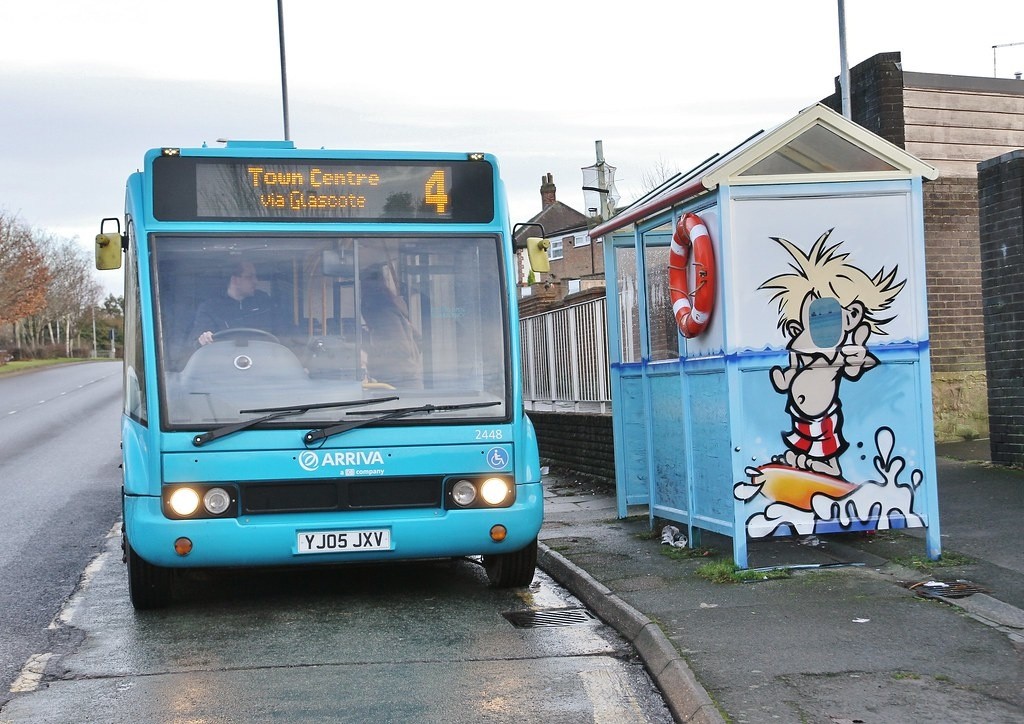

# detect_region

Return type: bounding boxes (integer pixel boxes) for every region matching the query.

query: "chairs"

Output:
[327,319,360,339]
[302,318,324,337]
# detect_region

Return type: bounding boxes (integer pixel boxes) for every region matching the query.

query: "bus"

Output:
[94,138,551,610]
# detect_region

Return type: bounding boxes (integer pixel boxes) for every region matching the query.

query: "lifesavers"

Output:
[668,213,715,337]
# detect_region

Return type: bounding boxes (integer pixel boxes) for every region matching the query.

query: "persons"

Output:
[184,260,272,352]
[358,262,424,393]
